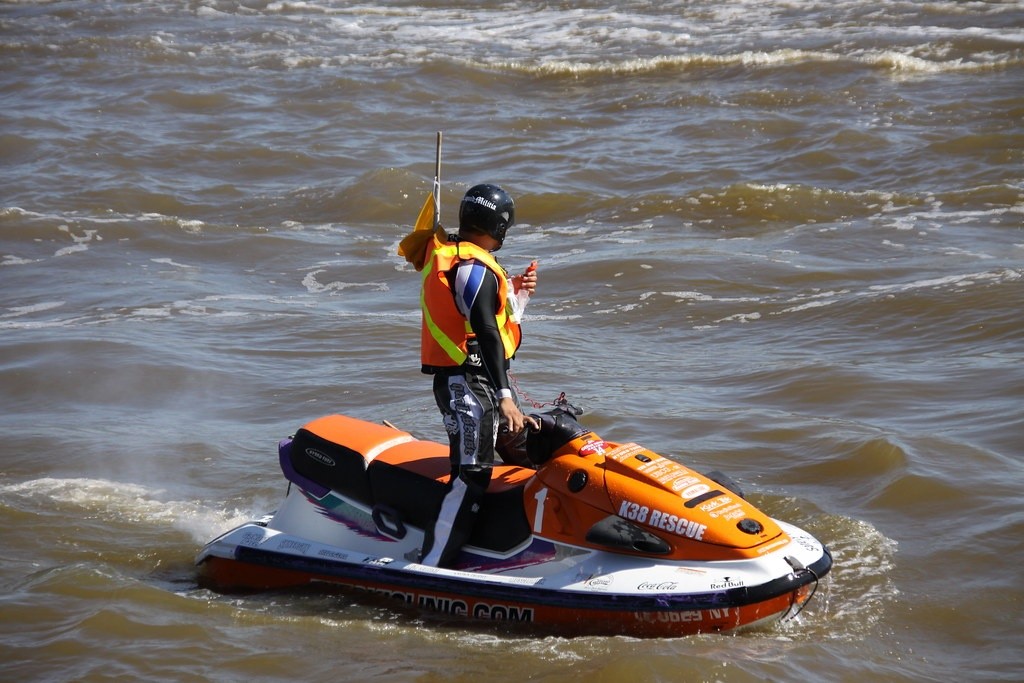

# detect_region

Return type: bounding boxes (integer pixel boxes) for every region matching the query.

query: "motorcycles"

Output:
[195,402,833,638]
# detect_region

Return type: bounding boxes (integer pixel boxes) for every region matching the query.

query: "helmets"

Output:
[460,183,515,241]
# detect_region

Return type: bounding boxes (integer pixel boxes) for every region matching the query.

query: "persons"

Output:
[418,184,539,569]
